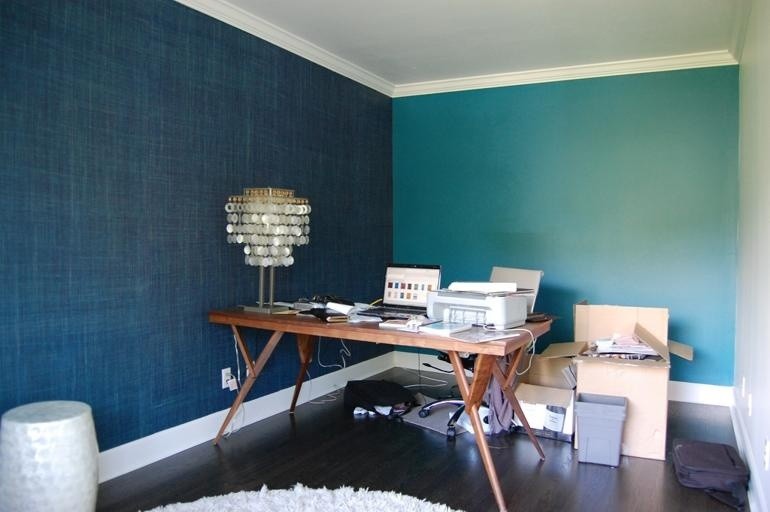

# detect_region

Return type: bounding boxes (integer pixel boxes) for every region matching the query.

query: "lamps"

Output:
[225,186,312,315]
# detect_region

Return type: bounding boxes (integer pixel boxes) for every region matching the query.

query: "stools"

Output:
[1,400,100,512]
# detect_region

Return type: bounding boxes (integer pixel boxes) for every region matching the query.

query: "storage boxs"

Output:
[537,322,670,460]
[572,300,694,360]
[514,354,576,443]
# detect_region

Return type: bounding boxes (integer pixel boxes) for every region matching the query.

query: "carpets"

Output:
[137,483,461,511]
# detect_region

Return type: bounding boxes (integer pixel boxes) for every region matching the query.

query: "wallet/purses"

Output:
[313,307,348,323]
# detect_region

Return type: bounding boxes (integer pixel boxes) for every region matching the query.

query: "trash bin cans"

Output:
[573,392,628,469]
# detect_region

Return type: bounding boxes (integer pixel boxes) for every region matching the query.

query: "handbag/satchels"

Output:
[345,379,417,416]
[671,437,749,509]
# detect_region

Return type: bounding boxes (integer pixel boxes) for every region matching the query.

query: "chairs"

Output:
[418,267,544,440]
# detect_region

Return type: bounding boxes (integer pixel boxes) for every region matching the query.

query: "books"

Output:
[379,315,425,328]
[450,326,520,344]
[417,320,472,336]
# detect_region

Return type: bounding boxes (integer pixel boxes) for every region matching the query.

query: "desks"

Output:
[207,303,562,512]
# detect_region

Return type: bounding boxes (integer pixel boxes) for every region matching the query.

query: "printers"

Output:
[427,289,527,330]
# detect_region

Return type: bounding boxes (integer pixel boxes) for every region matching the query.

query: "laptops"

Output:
[357,263,442,320]
